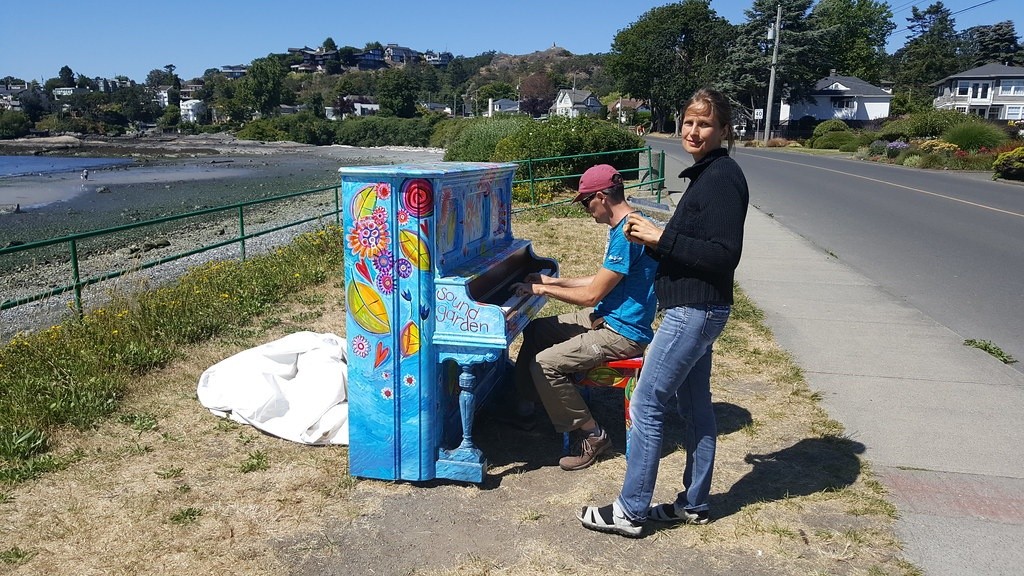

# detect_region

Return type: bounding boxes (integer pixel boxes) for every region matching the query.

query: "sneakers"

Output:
[559,426,611,470]
[487,402,537,430]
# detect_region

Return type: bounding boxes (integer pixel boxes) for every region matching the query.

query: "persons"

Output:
[575,89,749,536]
[488,164,658,471]
[636,125,645,137]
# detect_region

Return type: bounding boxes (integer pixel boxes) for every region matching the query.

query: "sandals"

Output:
[646,498,709,524]
[575,500,643,537]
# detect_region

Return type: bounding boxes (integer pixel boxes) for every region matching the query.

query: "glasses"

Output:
[581,192,608,207]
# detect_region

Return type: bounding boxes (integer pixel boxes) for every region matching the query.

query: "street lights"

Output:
[571,72,582,118]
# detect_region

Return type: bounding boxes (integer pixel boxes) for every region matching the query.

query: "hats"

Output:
[571,164,623,204]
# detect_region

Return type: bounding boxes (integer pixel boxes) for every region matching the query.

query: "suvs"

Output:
[733,125,755,138]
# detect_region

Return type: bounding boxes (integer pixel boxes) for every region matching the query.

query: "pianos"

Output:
[334,161,560,487]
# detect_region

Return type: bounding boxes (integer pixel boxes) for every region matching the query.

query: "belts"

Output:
[591,316,605,330]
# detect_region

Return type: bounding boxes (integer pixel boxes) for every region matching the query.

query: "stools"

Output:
[562,354,644,461]
